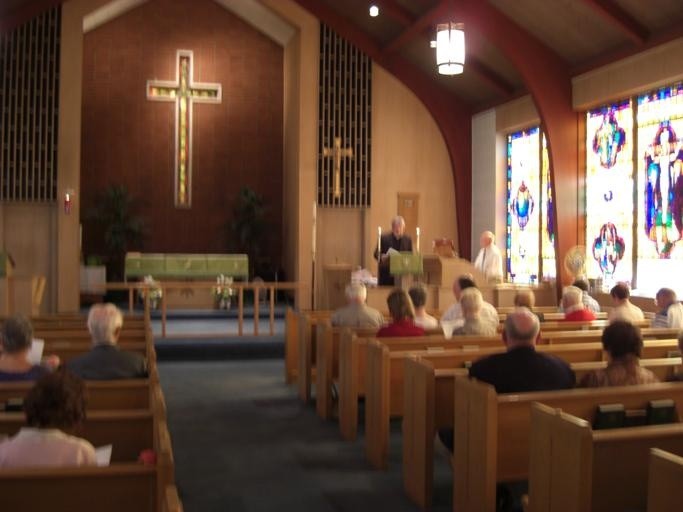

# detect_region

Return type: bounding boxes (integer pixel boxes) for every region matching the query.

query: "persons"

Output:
[373,215,413,286]
[580,318,662,388]
[472,230,505,284]
[0,370,99,468]
[435,286,498,468]
[328,277,681,343]
[466,308,576,510]
[0,309,62,381]
[60,300,150,380]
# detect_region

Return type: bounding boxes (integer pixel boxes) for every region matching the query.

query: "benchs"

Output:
[0,310,182,512]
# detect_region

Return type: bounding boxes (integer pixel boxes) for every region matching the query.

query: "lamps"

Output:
[428,1,466,75]
